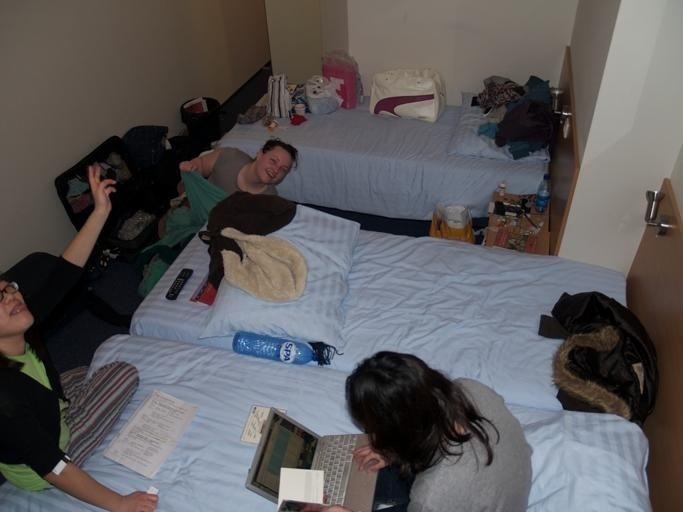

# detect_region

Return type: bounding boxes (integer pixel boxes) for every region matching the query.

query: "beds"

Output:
[129,219,628,411]
[0,333,652,512]
[216,92,550,221]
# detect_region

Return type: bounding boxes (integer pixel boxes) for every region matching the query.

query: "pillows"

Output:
[526,411,653,512]
[198,204,360,348]
[447,91,510,161]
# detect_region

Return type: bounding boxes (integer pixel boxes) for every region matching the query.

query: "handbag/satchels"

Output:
[205,191,296,235]
[369,67,445,124]
[265,52,364,120]
[430,205,475,243]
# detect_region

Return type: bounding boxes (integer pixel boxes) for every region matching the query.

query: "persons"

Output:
[0,163,161,512]
[177,137,299,251]
[323,350,534,511]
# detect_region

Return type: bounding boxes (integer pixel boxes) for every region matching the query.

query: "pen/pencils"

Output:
[524,214,538,228]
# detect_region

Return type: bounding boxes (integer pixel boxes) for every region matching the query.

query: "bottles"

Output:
[534,174,551,213]
[294,104,305,117]
[494,184,508,201]
[230,330,319,366]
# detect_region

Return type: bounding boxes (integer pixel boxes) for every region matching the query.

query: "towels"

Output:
[221,227,307,302]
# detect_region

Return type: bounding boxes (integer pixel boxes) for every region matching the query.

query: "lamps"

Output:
[551,88,564,114]
[645,191,668,236]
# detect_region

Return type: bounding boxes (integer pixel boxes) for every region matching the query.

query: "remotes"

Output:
[166,269,193,300]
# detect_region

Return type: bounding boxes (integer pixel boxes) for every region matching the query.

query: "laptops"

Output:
[246,408,378,512]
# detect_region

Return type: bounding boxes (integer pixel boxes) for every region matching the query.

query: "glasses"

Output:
[0,280,19,305]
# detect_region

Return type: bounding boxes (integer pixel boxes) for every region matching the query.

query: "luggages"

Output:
[54,136,180,264]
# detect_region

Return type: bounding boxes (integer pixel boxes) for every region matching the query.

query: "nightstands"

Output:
[486,192,550,255]
